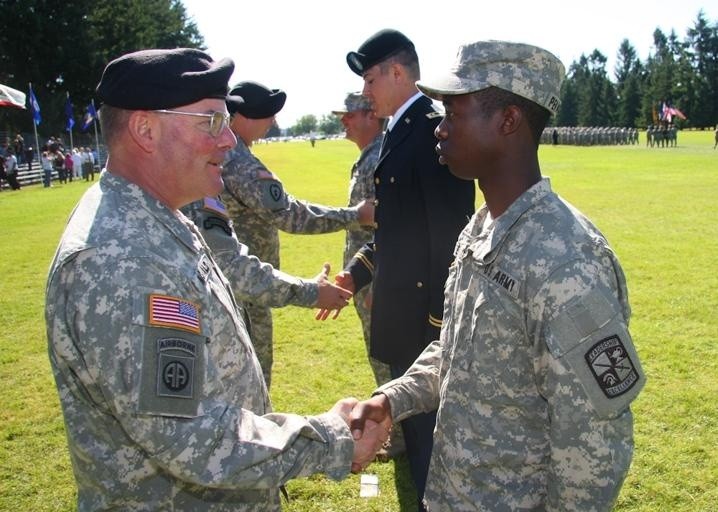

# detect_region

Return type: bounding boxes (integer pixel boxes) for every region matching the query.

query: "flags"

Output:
[29,87,42,125]
[658,98,686,121]
[64,96,75,135]
[79,103,96,129]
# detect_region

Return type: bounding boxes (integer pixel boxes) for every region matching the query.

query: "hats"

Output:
[346,29,412,76]
[414,40,566,119]
[225,94,245,114]
[229,81,286,119]
[96,48,235,110]
[331,92,372,114]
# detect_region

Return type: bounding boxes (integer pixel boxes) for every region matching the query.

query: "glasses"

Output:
[146,110,231,137]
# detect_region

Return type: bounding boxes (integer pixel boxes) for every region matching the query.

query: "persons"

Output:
[180,196,356,336]
[351,37,647,512]
[0,133,95,191]
[43,47,391,511]
[714,127,718,150]
[539,126,640,145]
[314,28,476,503]
[332,90,386,354]
[647,124,677,148]
[219,81,375,392]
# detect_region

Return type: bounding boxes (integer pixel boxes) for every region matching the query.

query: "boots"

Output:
[374,427,406,460]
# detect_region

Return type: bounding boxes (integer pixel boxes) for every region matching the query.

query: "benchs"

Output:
[0,131,109,191]
